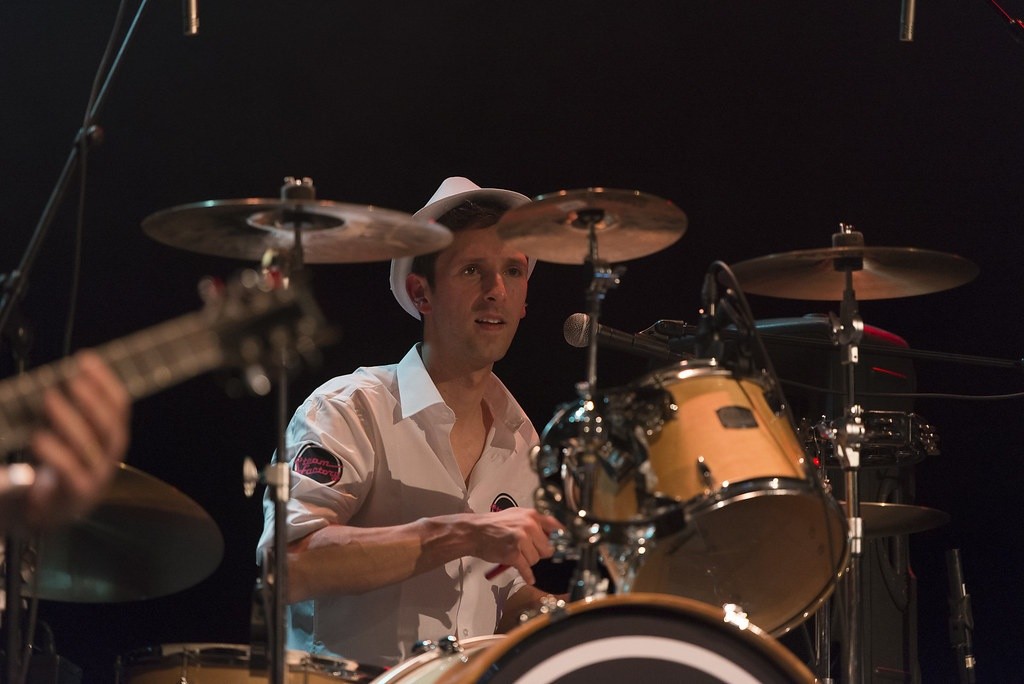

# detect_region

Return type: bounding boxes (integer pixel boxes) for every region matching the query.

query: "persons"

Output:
[0,348,131,526]
[257,174,577,684]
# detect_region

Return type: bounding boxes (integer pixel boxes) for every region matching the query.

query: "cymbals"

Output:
[838,502,948,539]
[917,423,940,456]
[140,198,454,265]
[0,460,225,604]
[716,246,979,301]
[495,188,688,264]
[867,417,896,439]
[724,316,835,331]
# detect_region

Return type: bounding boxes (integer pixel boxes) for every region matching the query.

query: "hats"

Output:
[389,177,538,320]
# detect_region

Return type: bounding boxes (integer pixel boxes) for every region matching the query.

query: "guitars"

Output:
[0,266,330,458]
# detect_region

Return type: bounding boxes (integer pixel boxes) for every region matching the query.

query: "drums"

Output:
[559,357,851,638]
[804,408,940,471]
[115,640,387,684]
[371,589,824,684]
